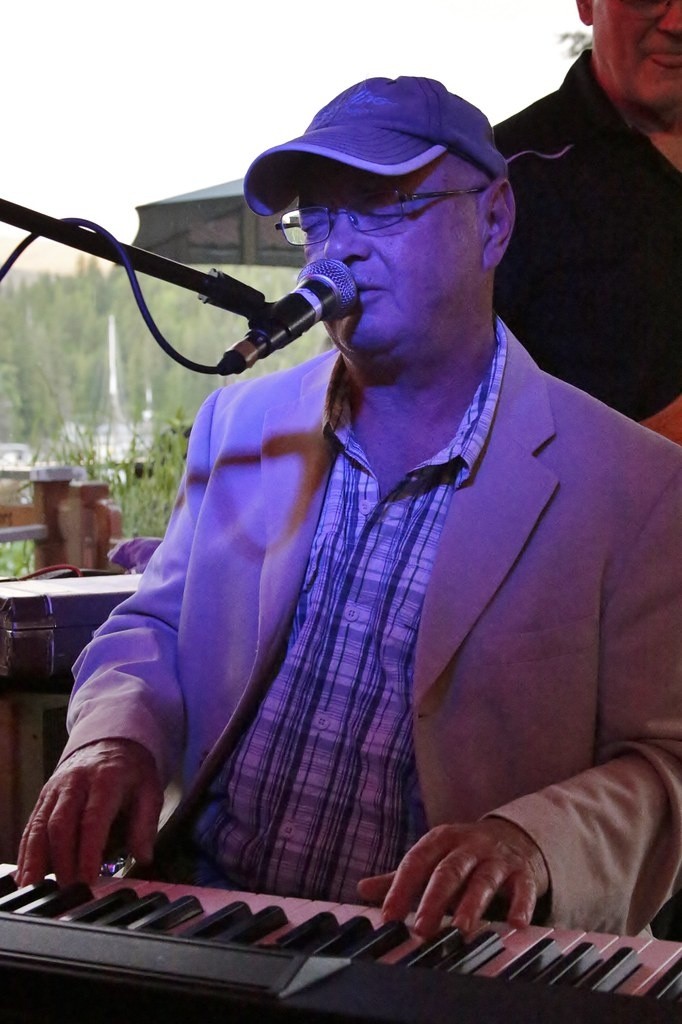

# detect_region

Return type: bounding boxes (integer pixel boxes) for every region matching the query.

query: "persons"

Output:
[15,0,681,934]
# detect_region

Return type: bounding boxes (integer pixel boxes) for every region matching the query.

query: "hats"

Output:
[242,76,508,216]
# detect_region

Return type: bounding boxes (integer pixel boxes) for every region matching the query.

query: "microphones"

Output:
[218,258,358,376]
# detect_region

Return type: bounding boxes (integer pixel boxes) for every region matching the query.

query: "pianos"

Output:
[1,860,681,1023]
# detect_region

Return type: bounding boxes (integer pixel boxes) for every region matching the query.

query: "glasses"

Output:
[273,188,487,247]
[618,0,672,18]
